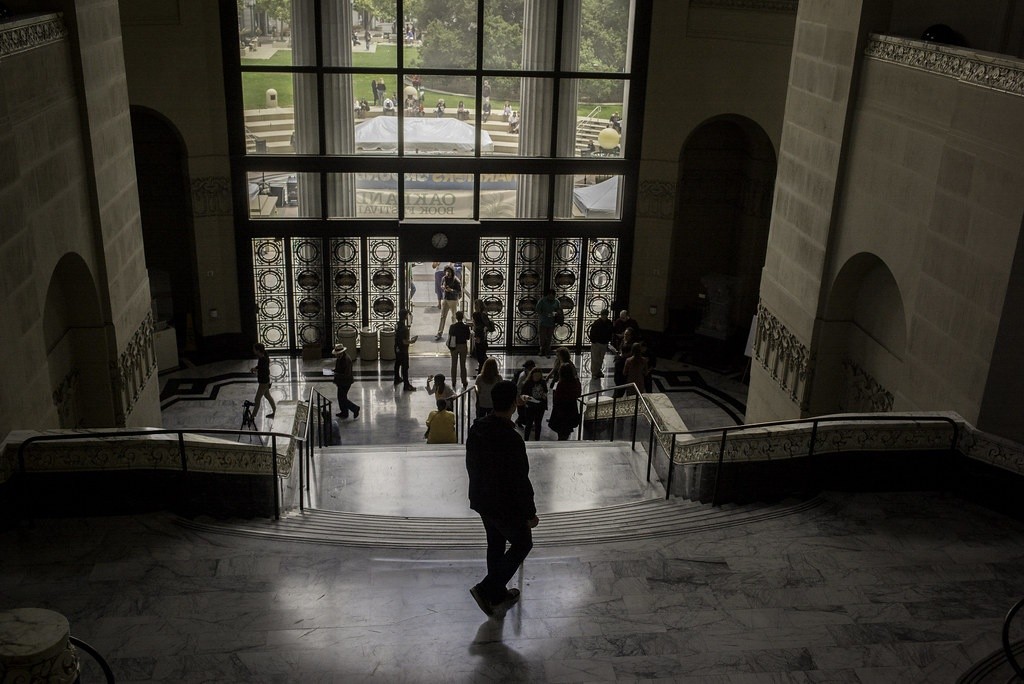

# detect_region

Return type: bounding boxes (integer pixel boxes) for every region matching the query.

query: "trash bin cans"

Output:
[380,331,395,360]
[339,329,357,362]
[360,330,378,360]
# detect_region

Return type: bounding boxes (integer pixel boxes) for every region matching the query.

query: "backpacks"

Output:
[511,370,527,385]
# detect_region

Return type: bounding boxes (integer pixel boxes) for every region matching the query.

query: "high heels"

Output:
[463,382,468,390]
[452,381,455,387]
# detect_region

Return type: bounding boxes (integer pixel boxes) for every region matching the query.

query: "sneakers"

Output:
[470,583,494,618]
[494,588,520,615]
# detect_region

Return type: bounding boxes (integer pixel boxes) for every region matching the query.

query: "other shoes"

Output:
[403,384,416,391]
[592,375,597,379]
[394,377,403,384]
[515,418,524,430]
[336,413,348,418]
[266,413,274,418]
[545,352,550,358]
[435,334,441,340]
[599,372,604,377]
[531,424,535,430]
[354,406,360,418]
[538,352,544,356]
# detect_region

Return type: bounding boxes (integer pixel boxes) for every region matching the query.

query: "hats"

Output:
[331,343,347,354]
[399,309,410,318]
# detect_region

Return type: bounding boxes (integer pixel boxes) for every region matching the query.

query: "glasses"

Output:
[621,316,626,319]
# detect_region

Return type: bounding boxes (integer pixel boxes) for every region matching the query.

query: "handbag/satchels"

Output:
[544,388,553,421]
[474,336,480,343]
[424,426,430,438]
[269,382,272,389]
[446,324,456,348]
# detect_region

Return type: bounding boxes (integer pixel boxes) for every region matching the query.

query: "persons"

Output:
[623,342,649,396]
[393,309,418,391]
[447,311,471,391]
[466,380,539,620]
[470,310,490,379]
[589,309,612,379]
[425,374,456,443]
[587,111,623,152]
[332,343,361,418]
[545,346,577,423]
[432,262,462,341]
[536,289,565,357]
[249,343,276,421]
[547,363,582,441]
[352,75,520,133]
[511,359,549,441]
[352,23,415,49]
[409,262,416,299]
[612,310,642,398]
[475,358,503,419]
